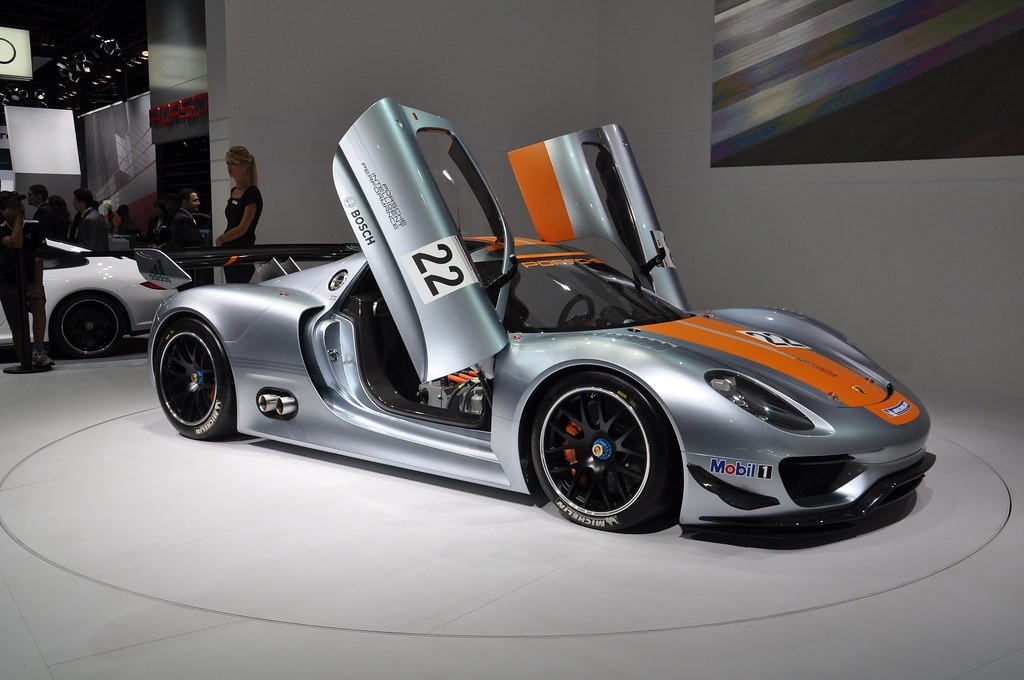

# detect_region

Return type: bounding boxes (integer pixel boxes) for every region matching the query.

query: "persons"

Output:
[215,145,264,285]
[116,205,138,232]
[27,185,111,251]
[0,190,57,367]
[146,189,205,250]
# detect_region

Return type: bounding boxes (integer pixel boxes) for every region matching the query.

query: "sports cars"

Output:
[1,238,179,360]
[133,96,931,539]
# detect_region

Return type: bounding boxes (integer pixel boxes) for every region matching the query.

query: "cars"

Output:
[159,212,211,241]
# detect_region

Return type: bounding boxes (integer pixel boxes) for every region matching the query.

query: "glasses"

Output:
[28,193,33,197]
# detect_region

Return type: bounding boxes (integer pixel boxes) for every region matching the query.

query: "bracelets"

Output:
[219,235,224,244]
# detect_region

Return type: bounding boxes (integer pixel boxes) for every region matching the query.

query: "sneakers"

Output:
[32,349,54,367]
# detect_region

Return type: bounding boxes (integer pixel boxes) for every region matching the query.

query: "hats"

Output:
[0,190,26,210]
